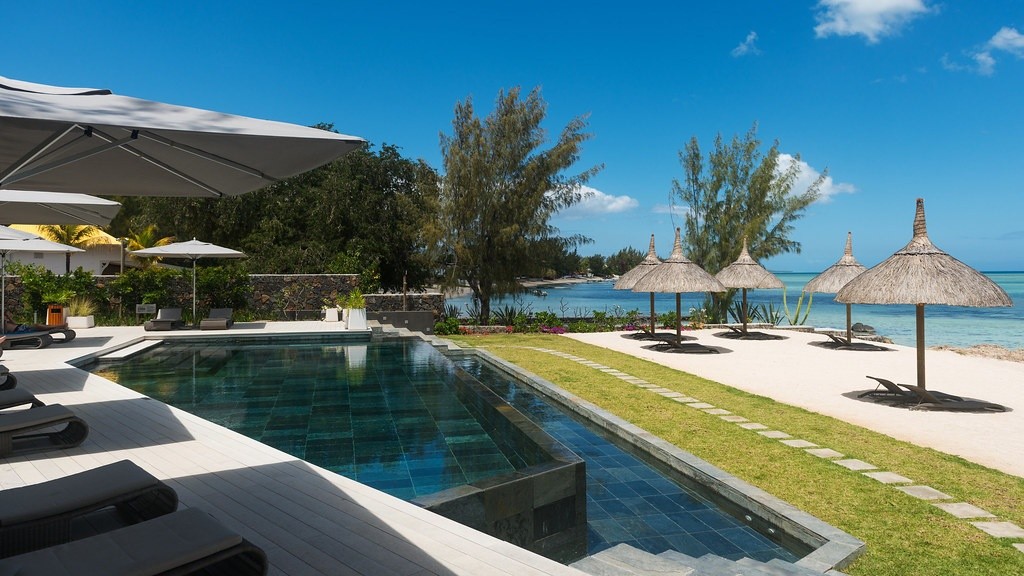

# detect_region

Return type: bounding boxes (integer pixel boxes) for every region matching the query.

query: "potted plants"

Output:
[345,285,368,331]
[320,289,347,322]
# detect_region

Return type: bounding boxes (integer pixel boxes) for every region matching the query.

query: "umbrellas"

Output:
[802,232,868,346]
[835,199,1012,403]
[633,228,728,347]
[613,232,664,338]
[712,234,784,337]
[2,73,367,358]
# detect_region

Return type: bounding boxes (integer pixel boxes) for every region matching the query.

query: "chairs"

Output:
[898,383,1006,412]
[0,504,269,576]
[50,328,76,344]
[0,459,180,560]
[200,308,233,330]
[1,330,53,349]
[630,327,889,354]
[857,375,964,403]
[0,364,91,458]
[143,307,185,331]
